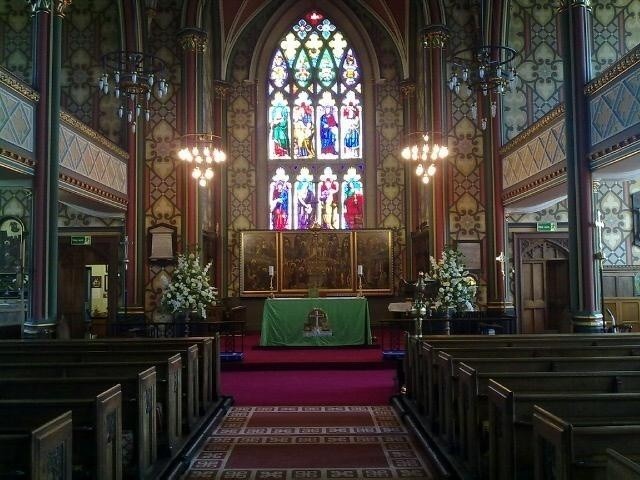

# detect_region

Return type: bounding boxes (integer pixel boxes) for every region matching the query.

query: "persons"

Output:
[343,105,359,156]
[320,105,339,155]
[297,179,317,229]
[343,177,363,229]
[270,179,289,230]
[271,104,289,156]
[293,104,315,158]
[320,178,339,229]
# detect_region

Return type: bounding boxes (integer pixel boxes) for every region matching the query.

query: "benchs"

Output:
[2,331,233,480]
[401,326,638,477]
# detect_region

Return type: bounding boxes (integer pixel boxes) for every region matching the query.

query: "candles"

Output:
[268,265,274,275]
[357,264,363,274]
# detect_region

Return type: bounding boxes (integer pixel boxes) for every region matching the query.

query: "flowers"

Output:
[413,252,479,320]
[157,249,219,320]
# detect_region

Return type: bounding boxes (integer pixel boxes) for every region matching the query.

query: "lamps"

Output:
[397,2,517,185]
[96,0,228,189]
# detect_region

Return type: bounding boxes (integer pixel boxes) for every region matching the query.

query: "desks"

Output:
[260,298,373,347]
